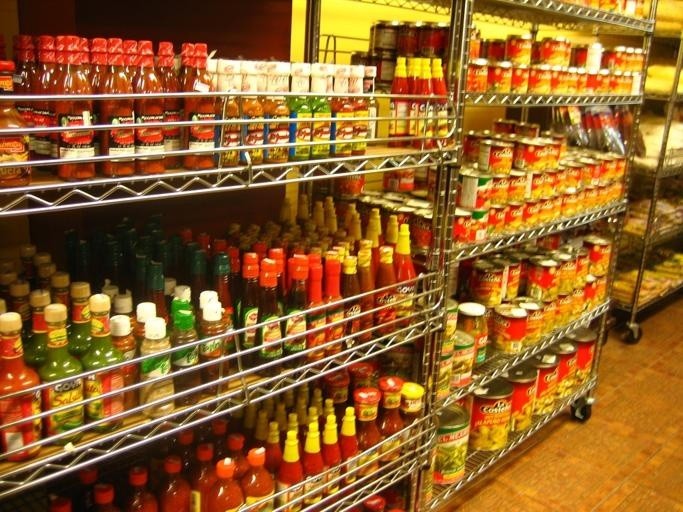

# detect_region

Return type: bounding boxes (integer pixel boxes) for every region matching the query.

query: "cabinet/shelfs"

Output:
[1,2,464,512]
[417,0,661,512]
[608,0,683,344]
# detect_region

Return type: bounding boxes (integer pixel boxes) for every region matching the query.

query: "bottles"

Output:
[133,301,157,357]
[164,277,176,311]
[323,398,336,424]
[255,270,282,377]
[432,58,447,148]
[332,65,354,157]
[147,256,170,323]
[217,59,240,167]
[389,56,409,148]
[213,253,235,368]
[124,466,159,511]
[316,234,348,259]
[14,36,35,170]
[242,448,276,512]
[225,432,248,481]
[303,406,321,441]
[62,212,232,253]
[251,408,269,448]
[365,218,382,270]
[193,250,207,309]
[172,311,201,406]
[326,64,335,106]
[113,294,136,332]
[242,404,258,441]
[236,263,258,370]
[52,272,71,323]
[350,363,373,387]
[302,420,323,504]
[158,455,193,512]
[196,290,218,338]
[0,298,6,315]
[413,57,423,149]
[354,387,381,485]
[401,382,426,450]
[102,285,118,301]
[158,42,182,169]
[283,263,306,366]
[298,193,310,220]
[123,40,138,83]
[350,64,368,155]
[349,213,361,255]
[294,396,307,448]
[324,259,343,354]
[140,316,175,418]
[172,285,192,305]
[0,312,41,462]
[31,35,55,172]
[184,43,217,171]
[378,376,401,473]
[395,223,415,326]
[50,497,71,512]
[82,293,125,432]
[177,429,196,477]
[112,315,139,417]
[131,41,164,176]
[242,61,264,165]
[325,208,339,233]
[376,245,395,333]
[326,372,350,433]
[325,197,333,219]
[20,243,37,281]
[201,302,229,395]
[385,215,398,273]
[360,240,374,250]
[190,443,218,512]
[275,402,287,452]
[342,256,361,348]
[208,418,230,460]
[30,252,52,289]
[87,38,108,153]
[265,61,290,165]
[307,261,325,361]
[233,218,316,263]
[38,303,83,446]
[0,270,17,311]
[24,290,51,368]
[57,35,95,181]
[408,58,414,144]
[290,63,312,161]
[69,281,92,362]
[49,36,66,175]
[311,388,324,430]
[258,62,268,107]
[262,397,276,420]
[357,250,374,342]
[87,482,121,512]
[11,279,31,346]
[321,413,341,495]
[369,208,385,246]
[208,459,244,512]
[0,257,16,272]
[79,38,91,78]
[98,38,135,178]
[345,202,357,232]
[149,423,174,493]
[283,393,296,417]
[364,65,379,146]
[133,249,148,309]
[287,412,302,457]
[414,58,432,149]
[310,63,331,158]
[177,42,194,91]
[168,298,193,348]
[0,61,32,189]
[39,263,55,299]
[275,430,303,511]
[263,421,283,474]
[78,466,98,512]
[299,383,309,407]
[339,407,357,484]
[312,201,325,228]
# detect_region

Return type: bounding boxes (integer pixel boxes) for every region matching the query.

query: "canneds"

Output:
[540,296,557,336]
[337,173,364,199]
[540,64,550,94]
[542,141,561,170]
[542,37,567,66]
[486,39,505,60]
[568,162,582,194]
[599,184,624,208]
[586,43,602,74]
[506,169,526,203]
[507,33,532,63]
[526,168,543,196]
[577,158,597,189]
[566,246,589,290]
[587,68,598,95]
[562,191,577,215]
[554,252,575,297]
[490,61,512,95]
[515,137,545,171]
[576,189,586,212]
[464,118,564,140]
[397,204,416,228]
[586,185,595,212]
[577,68,587,93]
[538,196,553,224]
[467,58,489,94]
[525,349,560,417]
[433,403,471,486]
[457,302,488,369]
[537,233,559,249]
[583,234,611,276]
[461,169,492,208]
[634,48,643,72]
[527,255,551,303]
[535,260,558,302]
[501,362,540,432]
[625,47,634,71]
[551,64,561,95]
[493,253,521,302]
[491,173,508,204]
[552,193,563,220]
[411,210,435,257]
[469,259,504,309]
[504,202,524,234]
[478,140,514,175]
[452,207,472,248]
[575,43,587,67]
[513,63,529,94]
[614,45,624,71]
[568,68,577,93]
[565,326,598,381]
[492,303,528,354]
[612,153,626,183]
[555,288,572,327]
[571,278,587,320]
[593,272,607,304]
[611,70,623,95]
[386,327,413,379]
[530,64,539,95]
[488,205,505,237]
[519,302,543,346]
[383,156,416,193]
[351,20,446,62]
[583,274,598,312]
[468,376,513,451]
[523,198,539,230]
[439,297,458,360]
[511,251,529,295]
[596,156,613,183]
[544,168,557,196]
[556,166,566,193]
[436,354,454,401]
[469,25,481,59]
[623,71,632,95]
[633,72,642,95]
[472,209,489,244]
[547,337,578,399]
[562,65,570,94]
[363,191,432,213]
[598,69,610,96]
[450,329,476,391]
